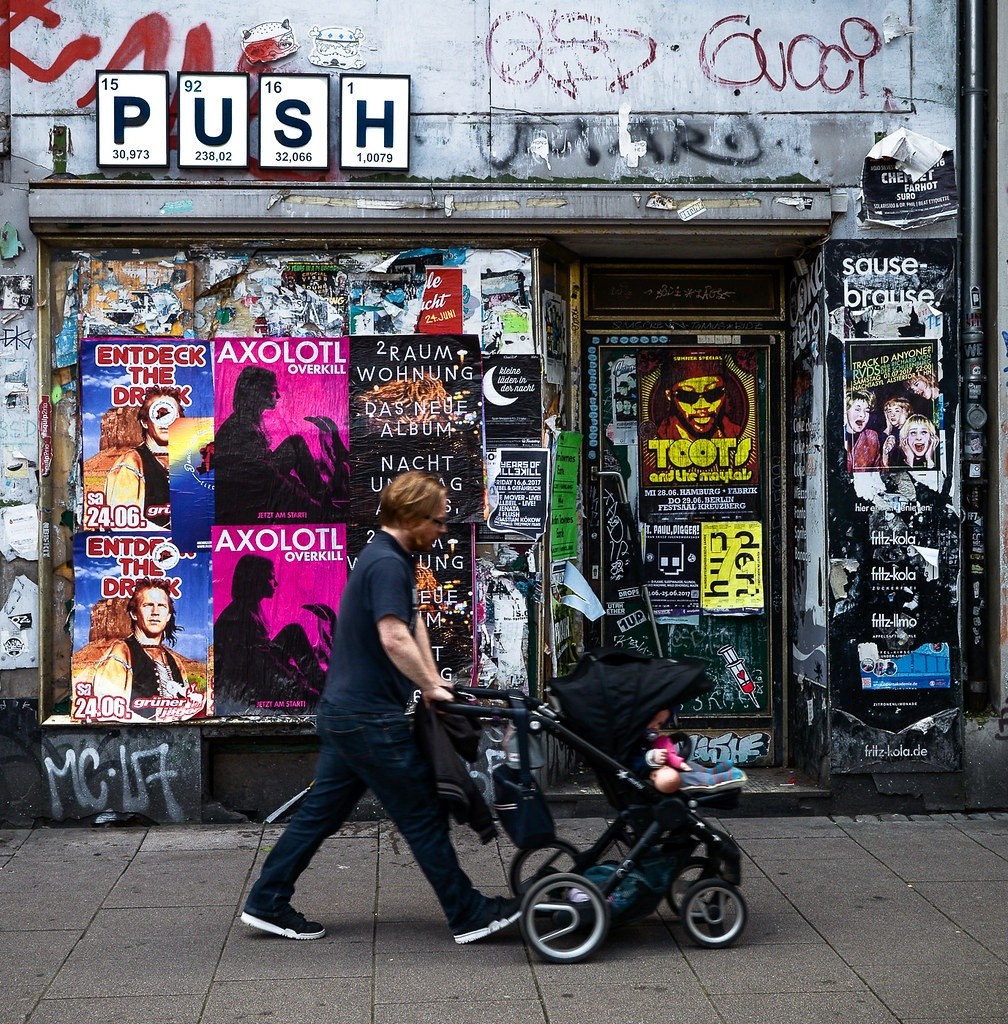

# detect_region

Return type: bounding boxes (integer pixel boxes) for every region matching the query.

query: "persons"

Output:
[846,371,940,472]
[93,577,191,723]
[215,365,345,524]
[105,386,185,532]
[239,473,524,944]
[214,554,327,714]
[639,708,742,793]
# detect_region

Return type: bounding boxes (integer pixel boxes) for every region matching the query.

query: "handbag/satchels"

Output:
[492,764,556,849]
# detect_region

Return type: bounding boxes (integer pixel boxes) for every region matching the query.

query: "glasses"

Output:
[421,515,449,529]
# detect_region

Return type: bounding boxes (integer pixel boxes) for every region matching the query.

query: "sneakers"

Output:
[445,889,522,945]
[241,900,326,939]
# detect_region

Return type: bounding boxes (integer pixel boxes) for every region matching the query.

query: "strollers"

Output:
[429,646,748,965]
